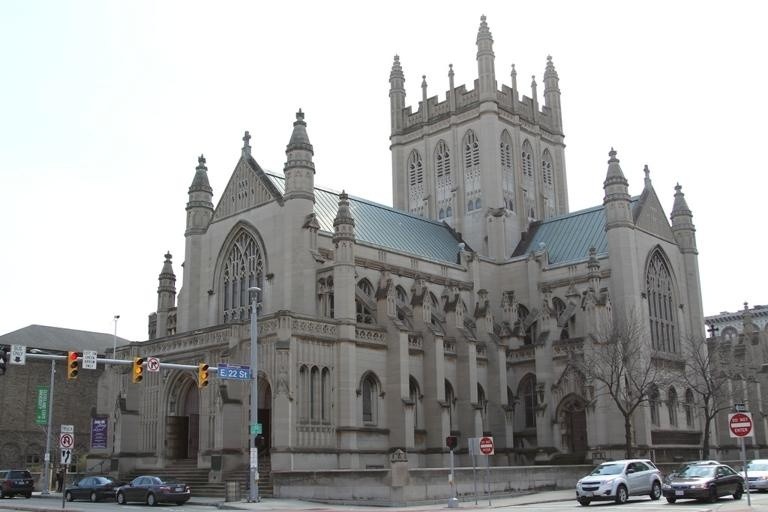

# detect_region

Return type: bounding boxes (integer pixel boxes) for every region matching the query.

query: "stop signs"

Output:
[728,412,754,438]
[479,437,494,456]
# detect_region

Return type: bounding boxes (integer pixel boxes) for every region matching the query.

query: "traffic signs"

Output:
[217,363,254,380]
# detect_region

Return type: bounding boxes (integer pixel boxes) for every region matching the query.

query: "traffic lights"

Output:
[133,356,144,384]
[67,351,80,380]
[199,363,209,388]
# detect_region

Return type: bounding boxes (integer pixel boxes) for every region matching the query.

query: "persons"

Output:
[56,468,65,493]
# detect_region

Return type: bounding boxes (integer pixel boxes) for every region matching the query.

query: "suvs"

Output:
[0,470,35,499]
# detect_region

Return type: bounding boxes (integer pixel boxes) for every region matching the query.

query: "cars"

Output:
[64,475,192,506]
[575,459,768,506]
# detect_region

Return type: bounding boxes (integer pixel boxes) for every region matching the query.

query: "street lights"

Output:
[30,349,56,494]
[247,286,262,503]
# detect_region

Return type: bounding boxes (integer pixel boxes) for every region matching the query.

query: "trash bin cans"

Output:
[225,480,241,502]
